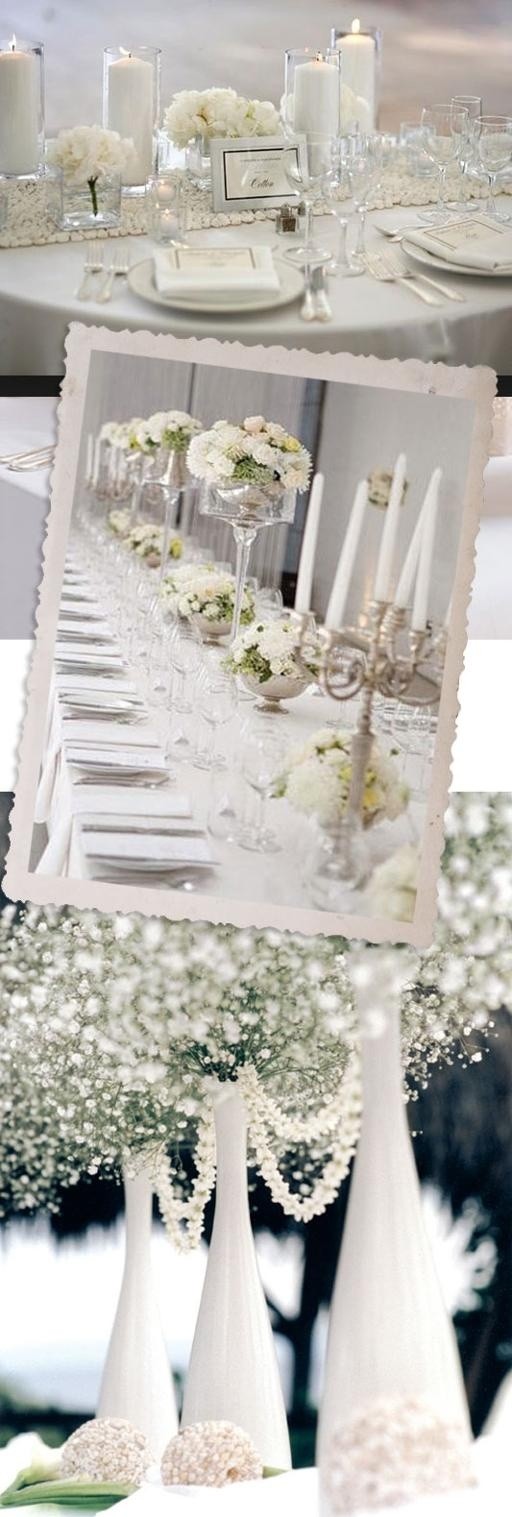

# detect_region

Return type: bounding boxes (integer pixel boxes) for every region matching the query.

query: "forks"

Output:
[88,861,217,891]
[362,248,439,309]
[96,244,130,301]
[73,235,105,301]
[375,242,465,301]
[367,220,434,237]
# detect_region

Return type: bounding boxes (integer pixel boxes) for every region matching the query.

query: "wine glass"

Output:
[389,698,439,800]
[51,507,295,859]
[397,93,511,224]
[282,130,385,278]
[323,646,368,731]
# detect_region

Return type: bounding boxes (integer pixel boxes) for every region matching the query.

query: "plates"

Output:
[129,259,306,316]
[402,235,510,278]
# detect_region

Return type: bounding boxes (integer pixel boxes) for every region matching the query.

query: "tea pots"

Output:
[302,800,375,912]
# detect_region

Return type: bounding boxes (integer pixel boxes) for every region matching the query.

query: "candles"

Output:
[335,17,378,138]
[0,30,42,179]
[280,52,338,160]
[286,455,447,640]
[104,50,149,164]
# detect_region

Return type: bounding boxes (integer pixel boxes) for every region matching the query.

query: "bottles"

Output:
[276,201,297,235]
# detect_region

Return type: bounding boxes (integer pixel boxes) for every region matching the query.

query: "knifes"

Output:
[301,263,333,327]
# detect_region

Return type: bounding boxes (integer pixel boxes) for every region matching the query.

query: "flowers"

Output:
[287,732,420,824]
[161,87,280,156]
[228,619,310,674]
[101,401,314,642]
[38,128,137,218]
[1,814,511,1246]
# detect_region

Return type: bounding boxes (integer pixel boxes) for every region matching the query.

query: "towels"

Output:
[0,1466,289,1508]
[154,247,280,303]
[46,545,224,897]
[408,216,510,270]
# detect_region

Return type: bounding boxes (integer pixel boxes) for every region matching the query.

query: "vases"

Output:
[181,1095,283,1481]
[180,130,250,191]
[306,808,379,854]
[50,171,123,232]
[72,1132,178,1474]
[336,1036,473,1502]
[236,663,312,716]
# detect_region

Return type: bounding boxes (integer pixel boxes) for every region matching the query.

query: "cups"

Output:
[146,173,184,246]
[101,42,166,198]
[182,534,319,632]
[42,166,126,233]
[1,34,50,180]
[186,141,212,191]
[279,44,342,196]
[329,23,383,131]
